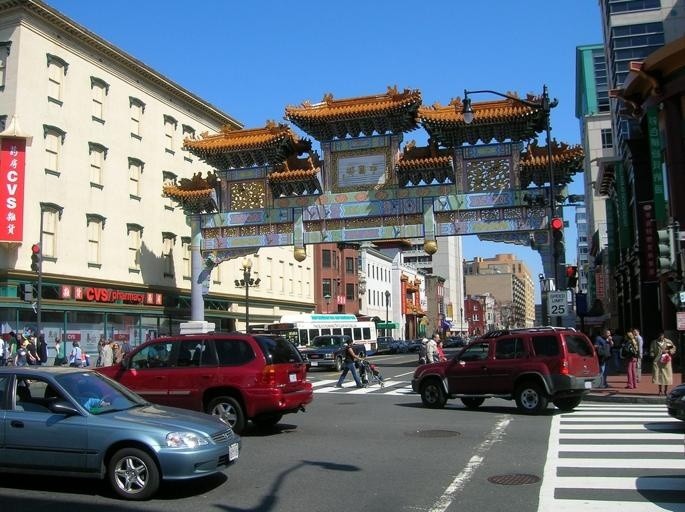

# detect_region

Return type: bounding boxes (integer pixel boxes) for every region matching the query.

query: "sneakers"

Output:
[661,353,670,364]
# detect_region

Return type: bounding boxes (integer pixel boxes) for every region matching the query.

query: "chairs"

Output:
[192,348,201,363]
[45,381,60,402]
[16,384,34,399]
[179,346,191,364]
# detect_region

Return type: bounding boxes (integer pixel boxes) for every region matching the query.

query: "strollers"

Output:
[359,347,385,390]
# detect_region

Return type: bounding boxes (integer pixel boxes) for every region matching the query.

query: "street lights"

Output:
[459,86,559,291]
[385,290,390,341]
[324,295,331,314]
[242,257,253,334]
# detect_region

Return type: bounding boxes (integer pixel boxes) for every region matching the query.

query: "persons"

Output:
[335,339,365,389]
[594,327,678,397]
[437,343,447,362]
[428,333,439,364]
[1,329,131,410]
[419,338,428,363]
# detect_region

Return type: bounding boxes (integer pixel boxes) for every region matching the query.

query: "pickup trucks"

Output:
[299,335,366,372]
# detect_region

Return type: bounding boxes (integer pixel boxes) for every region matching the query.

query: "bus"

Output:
[264,314,378,360]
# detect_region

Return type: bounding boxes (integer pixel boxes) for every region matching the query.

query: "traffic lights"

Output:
[551,218,564,252]
[31,243,40,272]
[567,265,576,287]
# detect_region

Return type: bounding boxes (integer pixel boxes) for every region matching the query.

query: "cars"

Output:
[388,336,463,353]
[0,365,240,501]
[667,384,685,421]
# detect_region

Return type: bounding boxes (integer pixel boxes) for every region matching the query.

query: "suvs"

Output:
[411,326,601,414]
[92,332,313,434]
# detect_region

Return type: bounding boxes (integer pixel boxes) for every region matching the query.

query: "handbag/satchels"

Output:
[75,360,82,366]
[62,356,68,364]
